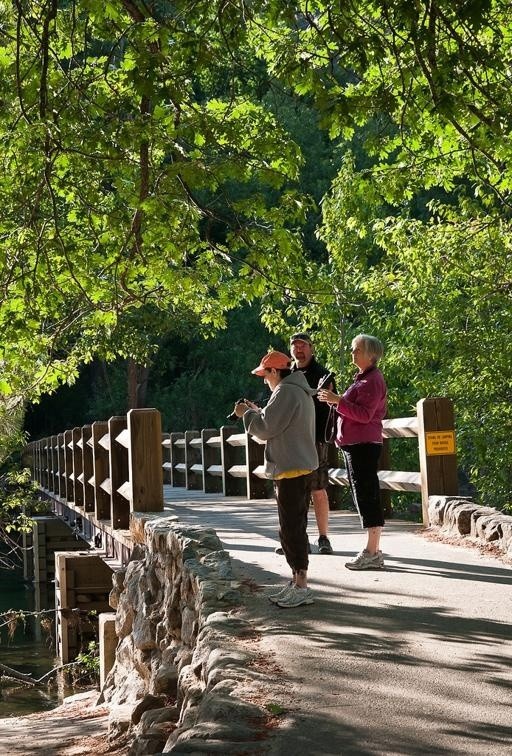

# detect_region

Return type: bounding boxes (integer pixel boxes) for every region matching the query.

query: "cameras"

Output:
[226,398,245,421]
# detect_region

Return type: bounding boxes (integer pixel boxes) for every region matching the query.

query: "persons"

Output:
[317,334,387,570]
[274,332,338,554]
[234,350,318,608]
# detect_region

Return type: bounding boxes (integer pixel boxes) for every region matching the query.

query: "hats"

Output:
[252,351,291,374]
[288,333,314,345]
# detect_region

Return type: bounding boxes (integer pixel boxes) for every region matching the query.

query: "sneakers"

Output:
[267,580,315,608]
[275,542,312,555]
[345,549,384,570]
[318,538,333,553]
